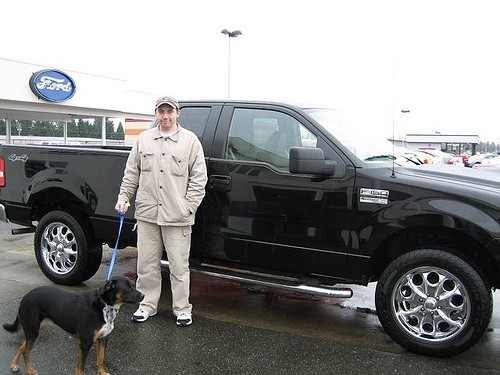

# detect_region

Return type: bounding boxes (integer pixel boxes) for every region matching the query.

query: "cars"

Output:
[401,146,500,177]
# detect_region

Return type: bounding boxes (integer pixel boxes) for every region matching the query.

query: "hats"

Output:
[155,96,179,111]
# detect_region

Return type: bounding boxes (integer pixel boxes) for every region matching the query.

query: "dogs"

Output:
[0,274,145,375]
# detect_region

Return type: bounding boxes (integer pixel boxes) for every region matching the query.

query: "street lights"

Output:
[222,30,242,101]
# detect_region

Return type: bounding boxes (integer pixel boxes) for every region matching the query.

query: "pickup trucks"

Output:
[0,102,500,360]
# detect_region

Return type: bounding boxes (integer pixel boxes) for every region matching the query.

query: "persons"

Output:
[114,96,209,327]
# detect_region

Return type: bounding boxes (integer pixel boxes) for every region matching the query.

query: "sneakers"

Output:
[130,305,157,323]
[176,313,193,327]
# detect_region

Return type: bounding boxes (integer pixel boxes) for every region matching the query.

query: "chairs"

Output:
[267,132,294,169]
[227,122,259,163]
